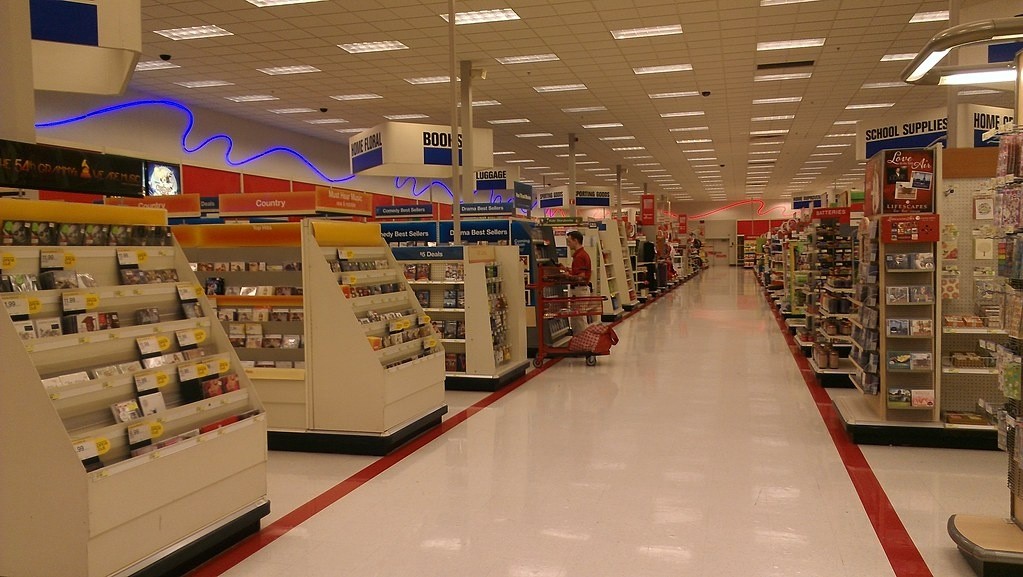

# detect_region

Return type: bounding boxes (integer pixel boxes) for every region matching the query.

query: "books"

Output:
[0,220,172,246]
[189,261,305,368]
[0,248,259,472]
[358,310,437,368]
[328,259,388,273]
[882,252,936,407]
[340,281,406,300]
[403,264,431,283]
[443,289,465,308]
[446,263,464,281]
[416,291,430,308]
[431,263,512,373]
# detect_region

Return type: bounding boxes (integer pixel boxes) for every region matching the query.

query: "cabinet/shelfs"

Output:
[832,142,1011,445]
[743,223,860,388]
[170,218,448,456]
[0,198,270,577]
[552,215,710,322]
[390,244,531,392]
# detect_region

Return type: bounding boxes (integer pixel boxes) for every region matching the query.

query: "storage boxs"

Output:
[850,237,880,395]
[188,259,431,369]
[404,264,466,372]
[0,220,260,474]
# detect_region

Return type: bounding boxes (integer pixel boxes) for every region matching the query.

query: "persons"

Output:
[563,231,592,337]
[89,225,107,247]
[36,222,56,246]
[9,221,32,246]
[112,225,131,246]
[690,233,700,255]
[63,224,84,246]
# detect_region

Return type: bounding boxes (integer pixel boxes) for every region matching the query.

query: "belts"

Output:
[570,281,590,286]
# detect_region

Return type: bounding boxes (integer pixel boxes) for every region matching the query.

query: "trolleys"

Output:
[525,262,612,369]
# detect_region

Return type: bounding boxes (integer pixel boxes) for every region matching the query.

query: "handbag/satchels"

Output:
[568,320,619,353]
